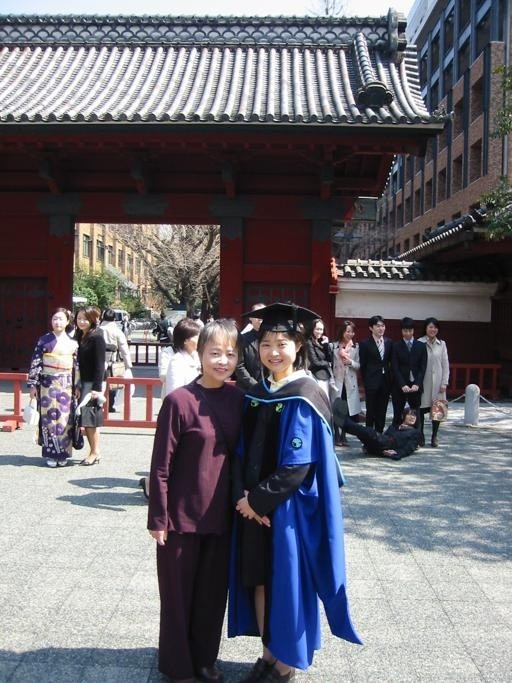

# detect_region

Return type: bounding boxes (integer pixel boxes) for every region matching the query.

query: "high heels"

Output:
[82,452,101,466]
[139,477,151,501]
[342,439,350,446]
[334,437,343,447]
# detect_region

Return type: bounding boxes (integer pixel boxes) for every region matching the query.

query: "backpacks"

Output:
[166,321,174,340]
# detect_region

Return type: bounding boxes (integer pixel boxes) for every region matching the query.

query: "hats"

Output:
[239,301,322,333]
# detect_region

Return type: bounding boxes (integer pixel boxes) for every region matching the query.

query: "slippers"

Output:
[46,457,58,468]
[58,461,67,466]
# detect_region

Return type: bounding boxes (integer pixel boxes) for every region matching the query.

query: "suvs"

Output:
[101,310,130,331]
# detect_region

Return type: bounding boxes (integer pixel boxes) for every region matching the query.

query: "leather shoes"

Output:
[239,656,273,683]
[196,664,225,683]
[419,434,426,447]
[261,659,295,682]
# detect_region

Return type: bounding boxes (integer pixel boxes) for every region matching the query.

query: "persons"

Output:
[100,310,132,414]
[229,303,363,683]
[74,306,106,466]
[26,308,82,467]
[361,314,396,434]
[330,322,361,448]
[120,315,133,337]
[332,398,422,461]
[394,318,428,451]
[414,316,449,451]
[303,318,336,399]
[153,312,174,345]
[234,304,267,391]
[138,319,201,501]
[148,318,249,682]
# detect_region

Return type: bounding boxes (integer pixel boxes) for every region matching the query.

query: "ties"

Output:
[407,341,413,352]
[376,338,384,359]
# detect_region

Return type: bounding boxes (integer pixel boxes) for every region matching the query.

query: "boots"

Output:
[429,433,438,447]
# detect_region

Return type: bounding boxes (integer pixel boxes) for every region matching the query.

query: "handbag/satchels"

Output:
[72,396,84,450]
[107,348,127,392]
[124,368,135,396]
[430,391,449,423]
